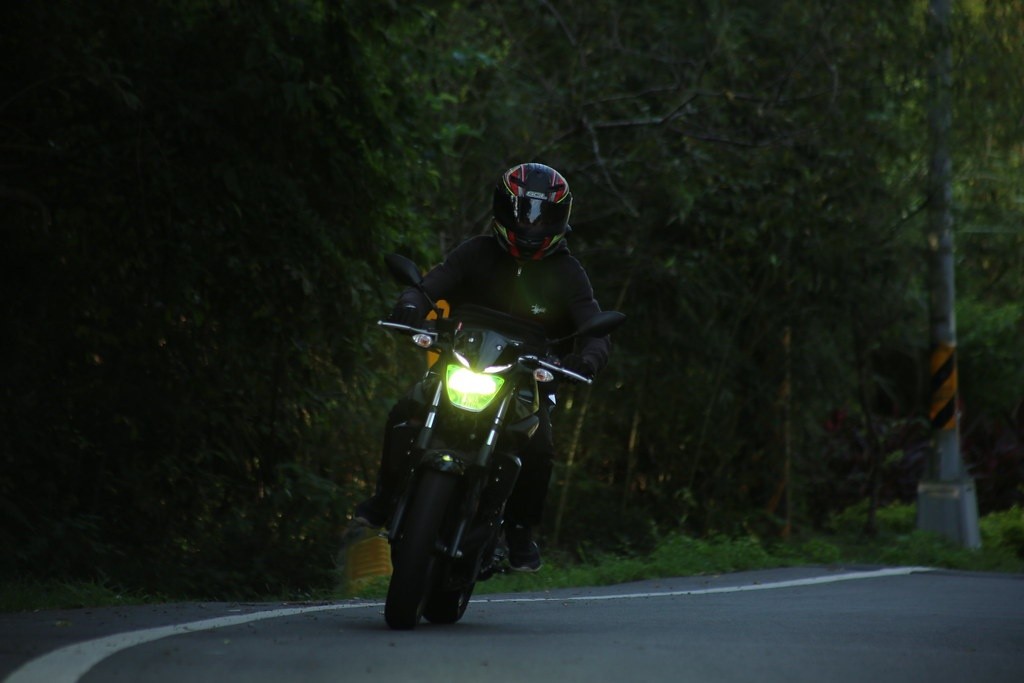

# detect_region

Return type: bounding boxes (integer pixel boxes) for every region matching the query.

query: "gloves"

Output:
[563,353,593,383]
[391,302,419,337]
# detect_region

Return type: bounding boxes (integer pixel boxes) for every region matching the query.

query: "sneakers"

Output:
[351,495,391,529]
[505,524,542,572]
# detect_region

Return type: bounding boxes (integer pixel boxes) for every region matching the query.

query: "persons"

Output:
[353,163,609,573]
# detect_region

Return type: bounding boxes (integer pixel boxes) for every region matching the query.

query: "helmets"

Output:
[489,163,573,265]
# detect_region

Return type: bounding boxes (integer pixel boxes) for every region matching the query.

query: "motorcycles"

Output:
[367,250,629,635]
